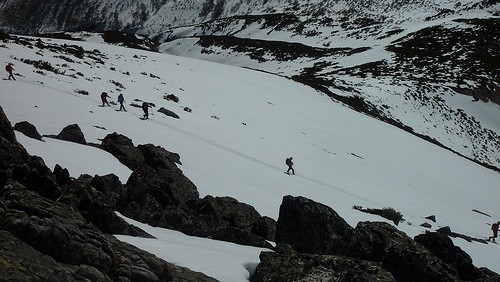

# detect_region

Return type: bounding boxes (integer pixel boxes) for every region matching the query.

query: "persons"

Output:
[101,92,111,107]
[489,220,500,243]
[142,102,150,119]
[118,94,127,112]
[285,157,295,175]
[5,62,16,81]
